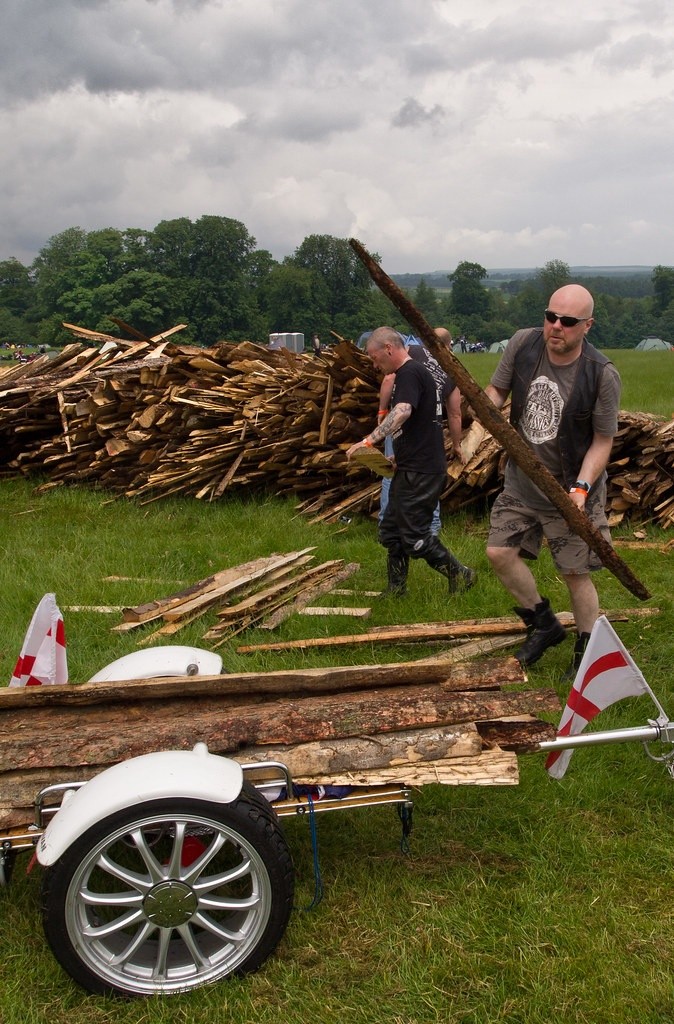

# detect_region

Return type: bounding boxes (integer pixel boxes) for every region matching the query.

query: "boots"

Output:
[513,596,565,666]
[563,630,593,682]
[424,543,477,596]
[379,556,408,598]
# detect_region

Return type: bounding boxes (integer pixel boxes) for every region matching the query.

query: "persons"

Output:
[346,326,471,600]
[378,328,466,536]
[14,349,22,359]
[5,342,8,350]
[460,335,467,353]
[471,342,483,353]
[312,335,321,356]
[468,284,621,682]
[40,346,46,354]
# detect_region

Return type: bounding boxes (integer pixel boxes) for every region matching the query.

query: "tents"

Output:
[453,343,471,353]
[635,336,670,351]
[489,339,508,353]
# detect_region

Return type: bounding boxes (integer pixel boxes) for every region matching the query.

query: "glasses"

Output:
[543,309,591,328]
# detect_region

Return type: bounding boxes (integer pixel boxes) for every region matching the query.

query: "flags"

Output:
[545,617,646,779]
[8,592,68,687]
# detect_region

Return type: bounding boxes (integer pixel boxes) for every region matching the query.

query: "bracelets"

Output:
[363,438,370,447]
[378,409,388,414]
[569,480,591,497]
